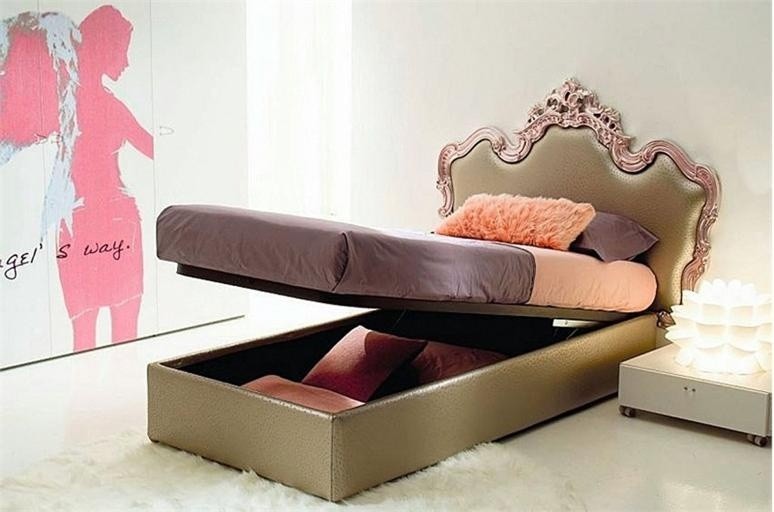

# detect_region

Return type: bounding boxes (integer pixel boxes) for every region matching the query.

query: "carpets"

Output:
[0,425,575,512]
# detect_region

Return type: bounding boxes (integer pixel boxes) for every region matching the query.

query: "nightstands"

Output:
[616,344,770,450]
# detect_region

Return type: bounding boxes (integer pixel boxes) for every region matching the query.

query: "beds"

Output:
[147,80,720,505]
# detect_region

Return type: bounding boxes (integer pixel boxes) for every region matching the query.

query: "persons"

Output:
[53,2,154,356]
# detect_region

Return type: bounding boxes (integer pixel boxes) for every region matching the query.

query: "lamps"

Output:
[662,275,770,374]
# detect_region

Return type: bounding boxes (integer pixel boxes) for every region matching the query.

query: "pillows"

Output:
[573,206,658,268]
[391,338,508,392]
[299,322,429,401]
[434,193,594,252]
[239,373,369,415]
